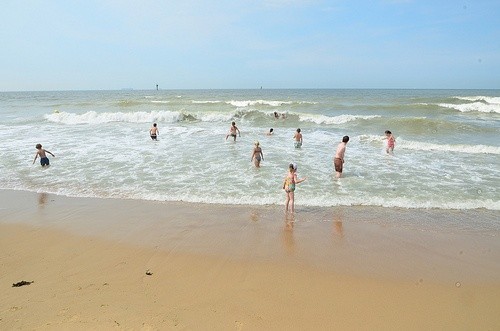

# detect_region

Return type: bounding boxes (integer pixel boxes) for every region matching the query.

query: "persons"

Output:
[32,144,55,167]
[333,136,349,178]
[268,128,273,134]
[251,141,264,167]
[225,122,241,141]
[282,162,306,214]
[274,111,287,119]
[383,131,395,153]
[293,128,302,149]
[149,123,159,139]
